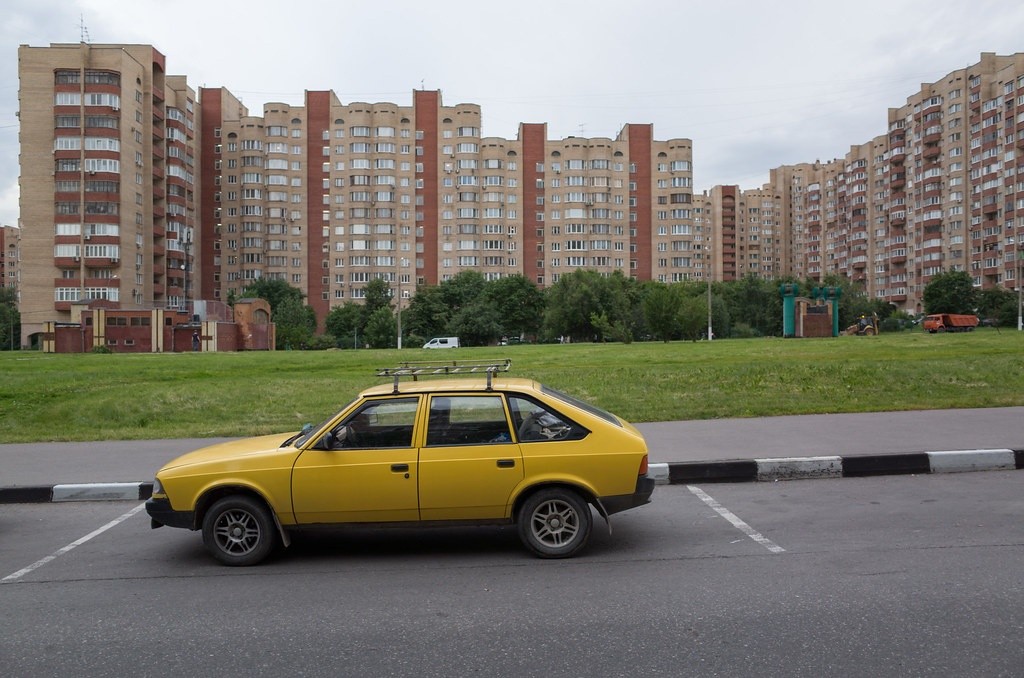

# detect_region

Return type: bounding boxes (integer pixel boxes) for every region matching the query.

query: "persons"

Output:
[191,330,202,351]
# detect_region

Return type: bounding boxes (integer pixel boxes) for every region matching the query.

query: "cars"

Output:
[640,334,653,342]
[137,358,654,565]
[912,316,925,325]
[497,339,508,346]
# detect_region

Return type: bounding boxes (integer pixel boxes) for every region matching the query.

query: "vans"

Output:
[423,337,461,350]
[543,338,561,344]
[508,336,520,344]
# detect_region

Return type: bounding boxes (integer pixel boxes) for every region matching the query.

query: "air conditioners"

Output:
[137,242,142,248]
[111,258,118,263]
[281,217,286,221]
[84,235,90,240]
[264,152,267,155]
[132,127,135,131]
[133,288,138,295]
[113,106,118,110]
[232,247,237,251]
[72,256,79,261]
[136,265,140,269]
[89,170,94,174]
[264,186,267,188]
[339,103,1024,287]
[136,161,144,167]
[289,217,294,221]
[171,212,176,216]
[169,138,175,141]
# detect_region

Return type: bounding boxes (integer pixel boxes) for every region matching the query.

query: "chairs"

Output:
[427,396,451,446]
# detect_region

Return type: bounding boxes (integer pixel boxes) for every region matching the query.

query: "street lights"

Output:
[108,275,117,308]
[706,246,713,341]
[397,257,404,350]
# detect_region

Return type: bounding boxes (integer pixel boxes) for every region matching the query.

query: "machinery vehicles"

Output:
[923,313,979,333]
[840,311,880,337]
